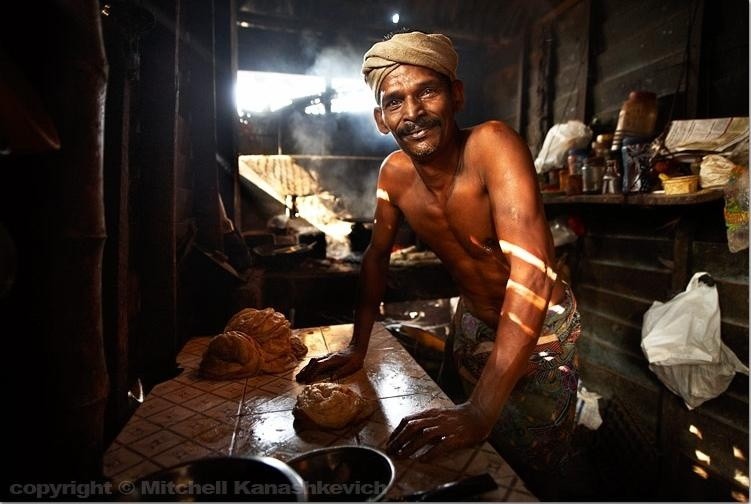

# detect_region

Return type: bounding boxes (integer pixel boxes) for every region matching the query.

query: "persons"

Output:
[295,29,585,502]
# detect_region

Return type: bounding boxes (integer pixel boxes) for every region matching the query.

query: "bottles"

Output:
[571,91,658,193]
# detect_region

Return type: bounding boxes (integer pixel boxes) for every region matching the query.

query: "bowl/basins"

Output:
[108,456,306,502]
[285,446,396,501]
